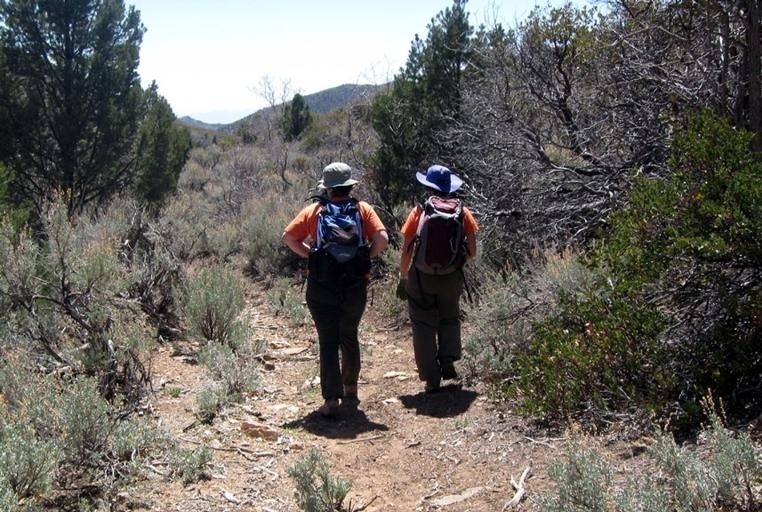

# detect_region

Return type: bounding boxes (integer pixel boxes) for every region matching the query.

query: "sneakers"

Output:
[425,353,457,391]
[319,397,360,416]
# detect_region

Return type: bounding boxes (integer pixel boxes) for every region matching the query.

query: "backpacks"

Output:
[310,198,368,263]
[407,196,470,276]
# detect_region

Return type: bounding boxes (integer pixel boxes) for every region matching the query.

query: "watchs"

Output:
[398,272,408,280]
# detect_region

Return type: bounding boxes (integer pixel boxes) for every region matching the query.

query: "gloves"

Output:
[396,279,408,301]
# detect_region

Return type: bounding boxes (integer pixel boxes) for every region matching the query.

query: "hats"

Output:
[416,165,463,193]
[316,162,360,189]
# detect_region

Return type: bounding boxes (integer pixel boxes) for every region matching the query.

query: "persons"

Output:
[282,162,390,417]
[396,164,480,392]
